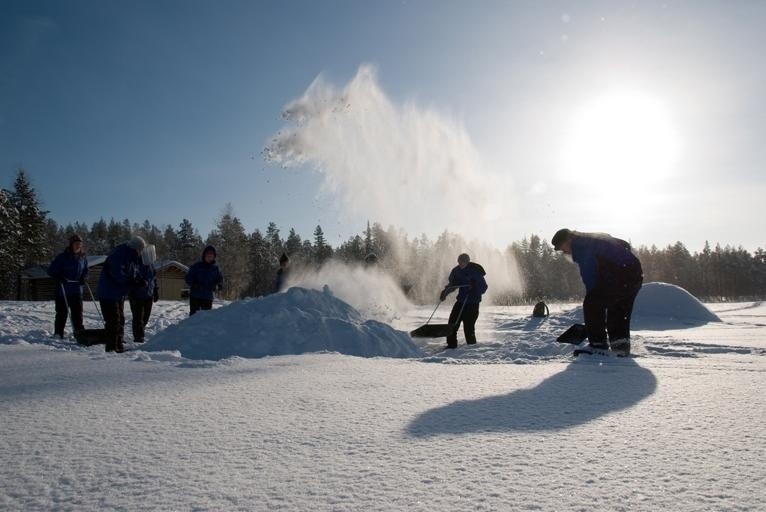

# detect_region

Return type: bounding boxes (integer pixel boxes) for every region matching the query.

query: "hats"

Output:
[365,253,381,262]
[69,234,82,246]
[551,229,577,250]
[457,253,470,264]
[204,246,218,258]
[279,253,288,266]
[128,236,147,250]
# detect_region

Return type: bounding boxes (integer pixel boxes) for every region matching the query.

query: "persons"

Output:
[440,253,487,349]
[184,245,224,316]
[551,228,643,358]
[47,234,88,340]
[127,246,159,343]
[96,236,145,354]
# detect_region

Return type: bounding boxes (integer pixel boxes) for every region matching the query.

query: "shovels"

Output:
[558,324,590,344]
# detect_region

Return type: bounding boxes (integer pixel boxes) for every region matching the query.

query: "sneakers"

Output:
[574,343,607,357]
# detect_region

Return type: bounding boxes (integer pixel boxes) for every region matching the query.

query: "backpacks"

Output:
[533,301,549,317]
